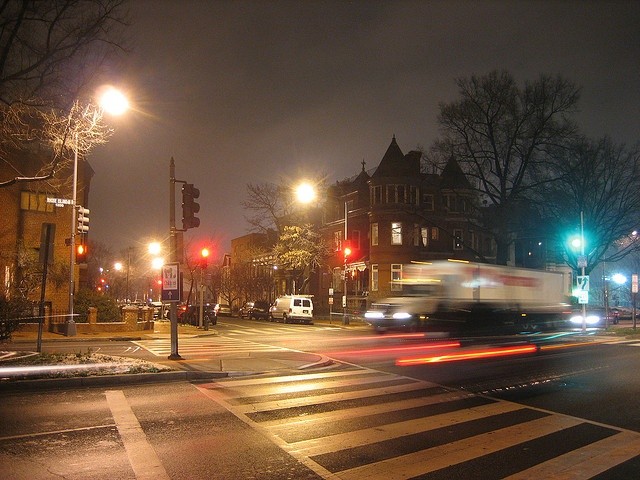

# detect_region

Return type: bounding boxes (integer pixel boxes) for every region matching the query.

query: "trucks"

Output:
[364,259,573,332]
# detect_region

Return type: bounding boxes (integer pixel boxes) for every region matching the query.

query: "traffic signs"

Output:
[160,262,180,303]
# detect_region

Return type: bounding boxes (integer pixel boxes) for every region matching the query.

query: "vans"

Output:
[268,293,314,324]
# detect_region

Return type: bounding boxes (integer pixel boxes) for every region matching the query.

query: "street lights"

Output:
[342,247,351,325]
[197,248,210,329]
[63,87,130,336]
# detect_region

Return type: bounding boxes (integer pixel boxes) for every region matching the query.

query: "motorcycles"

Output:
[192,306,217,331]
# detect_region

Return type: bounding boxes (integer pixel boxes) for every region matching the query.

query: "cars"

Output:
[129,299,189,323]
[210,303,231,317]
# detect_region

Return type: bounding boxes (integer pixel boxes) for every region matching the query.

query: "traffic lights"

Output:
[174,180,200,232]
[74,204,90,236]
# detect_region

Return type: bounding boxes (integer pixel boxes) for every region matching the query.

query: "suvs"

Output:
[238,301,271,321]
